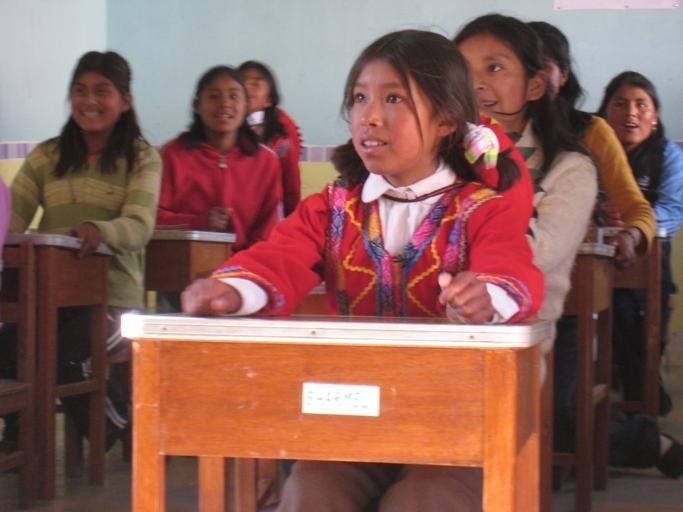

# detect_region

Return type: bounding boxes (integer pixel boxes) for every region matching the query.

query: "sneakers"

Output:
[660,388,672,415]
[656,432,683,477]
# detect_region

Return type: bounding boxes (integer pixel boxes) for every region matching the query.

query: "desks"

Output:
[1,226,671,512]
